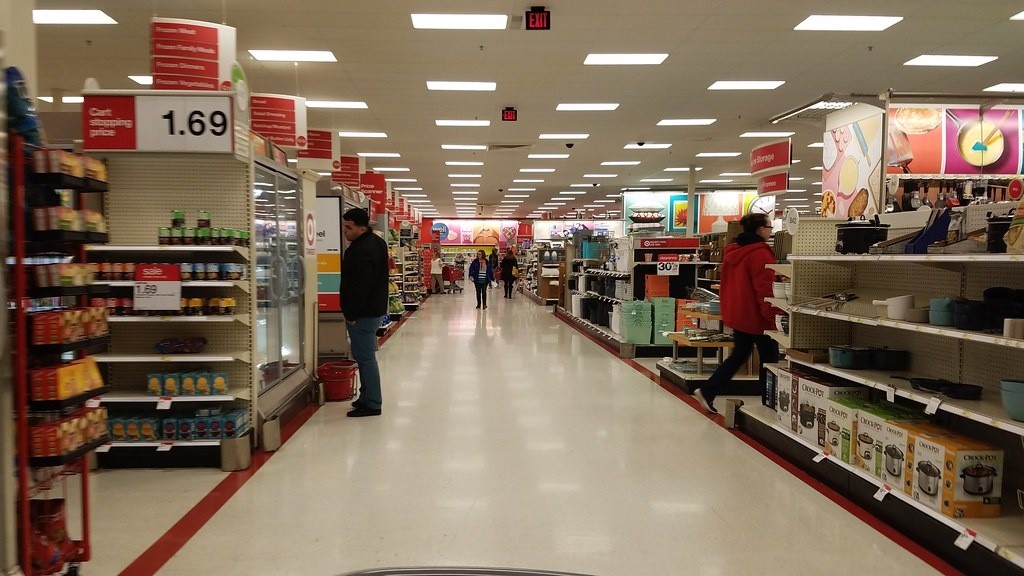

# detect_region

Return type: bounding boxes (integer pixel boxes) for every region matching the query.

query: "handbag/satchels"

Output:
[511,266,519,277]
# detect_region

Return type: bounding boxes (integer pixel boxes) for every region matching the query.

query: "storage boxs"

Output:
[726,221,744,242]
[760,369,1007,520]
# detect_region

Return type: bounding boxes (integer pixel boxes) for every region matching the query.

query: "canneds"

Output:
[158,209,250,247]
[91,297,236,315]
[89,263,246,282]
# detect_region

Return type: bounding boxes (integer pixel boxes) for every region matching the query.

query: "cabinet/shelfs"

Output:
[8,139,111,576]
[54,144,439,452]
[522,237,760,395]
[763,202,1024,576]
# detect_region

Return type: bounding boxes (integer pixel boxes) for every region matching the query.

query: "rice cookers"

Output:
[780,391,790,412]
[884,444,904,476]
[835,215,890,254]
[857,432,876,460]
[961,464,998,495]
[826,421,840,446]
[916,461,941,495]
[799,401,815,428]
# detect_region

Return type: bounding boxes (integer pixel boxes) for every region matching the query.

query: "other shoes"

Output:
[476,306,481,308]
[693,387,718,415]
[347,398,381,417]
[440,292,446,294]
[483,305,487,309]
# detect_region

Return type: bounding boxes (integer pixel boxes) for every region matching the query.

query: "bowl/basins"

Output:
[773,282,791,299]
[1000,380,1024,422]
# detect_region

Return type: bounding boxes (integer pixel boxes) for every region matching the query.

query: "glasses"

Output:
[763,226,774,230]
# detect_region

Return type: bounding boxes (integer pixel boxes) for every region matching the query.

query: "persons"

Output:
[694,213,779,414]
[564,223,584,238]
[500,250,518,298]
[430,251,446,294]
[339,208,389,416]
[489,248,498,288]
[551,222,561,248]
[468,250,495,309]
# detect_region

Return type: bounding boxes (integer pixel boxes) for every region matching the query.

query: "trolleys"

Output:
[437,265,464,295]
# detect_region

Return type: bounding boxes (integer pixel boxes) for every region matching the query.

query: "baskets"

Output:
[651,297,676,345]
[675,299,699,346]
[644,274,670,298]
[620,297,652,345]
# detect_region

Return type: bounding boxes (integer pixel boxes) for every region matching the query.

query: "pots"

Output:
[946,109,1004,166]
[919,384,982,400]
[890,375,943,393]
[872,295,929,323]
[929,297,953,326]
[986,208,1017,253]
